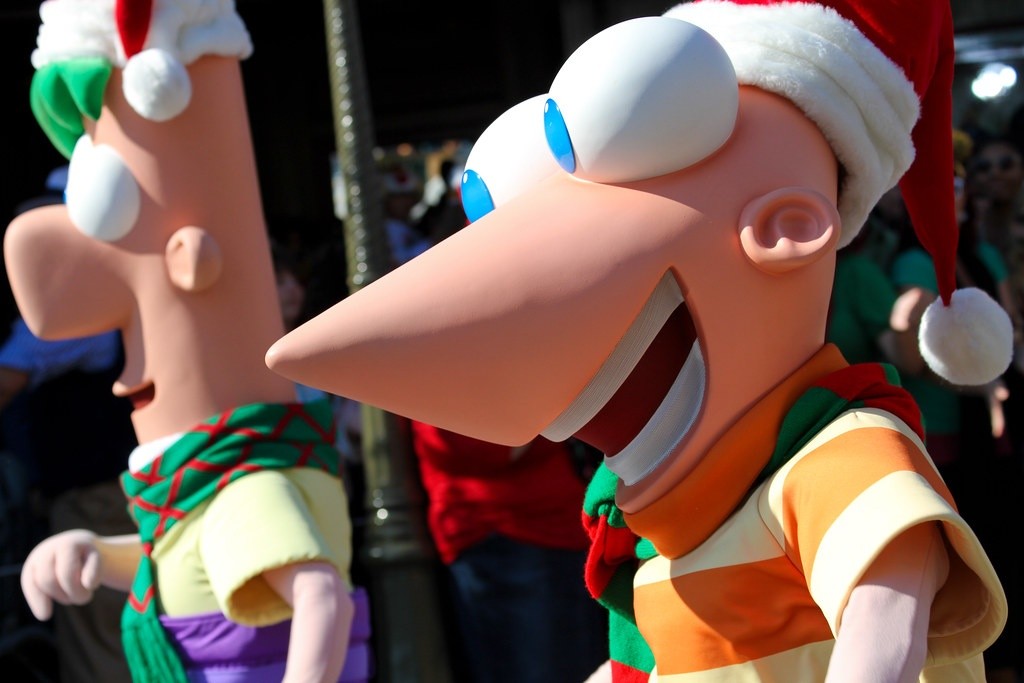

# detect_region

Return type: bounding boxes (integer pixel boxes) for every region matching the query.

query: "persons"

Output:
[262,1,1014,683]
[0,0,372,683]
[830,104,1024,683]
[410,421,609,683]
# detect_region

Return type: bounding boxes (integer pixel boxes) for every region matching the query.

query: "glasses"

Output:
[974,157,1019,174]
